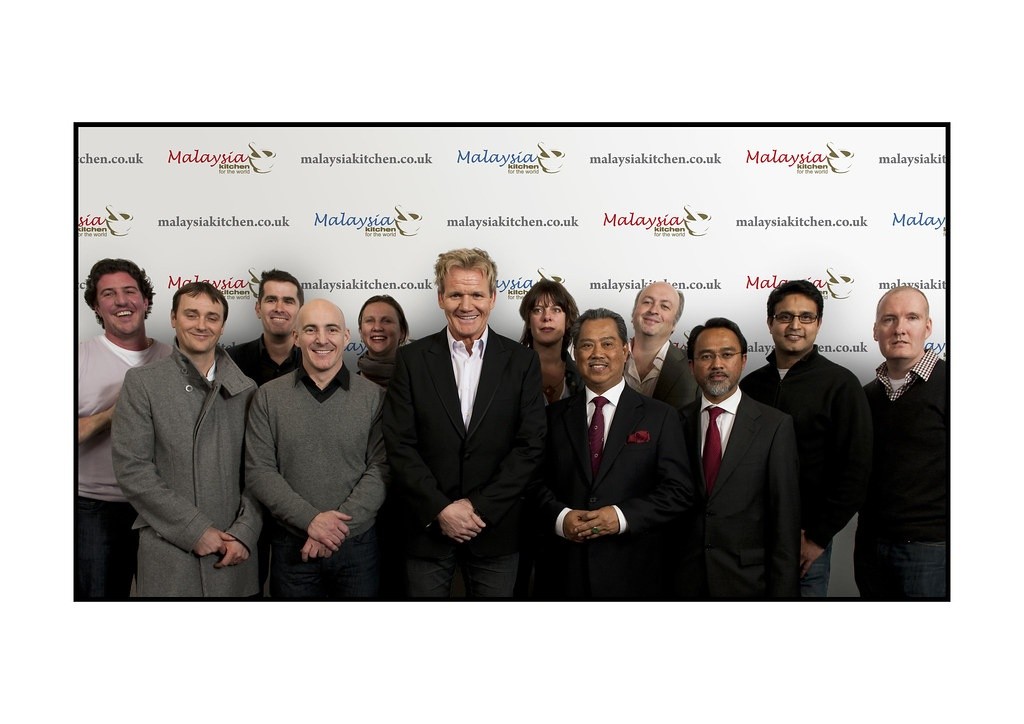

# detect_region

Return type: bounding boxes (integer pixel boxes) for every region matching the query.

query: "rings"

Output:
[592,527,599,534]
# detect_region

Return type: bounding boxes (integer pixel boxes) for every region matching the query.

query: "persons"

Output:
[223,270,302,388]
[78,259,174,597]
[244,298,390,599]
[739,280,872,597]
[623,283,702,408]
[357,295,408,391]
[530,308,693,602]
[520,281,585,406]
[382,249,548,598]
[678,318,801,601]
[853,287,946,598]
[110,282,262,597]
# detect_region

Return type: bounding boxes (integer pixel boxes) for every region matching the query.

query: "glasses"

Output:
[771,312,819,324]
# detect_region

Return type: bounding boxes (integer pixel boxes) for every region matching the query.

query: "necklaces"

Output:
[543,376,564,396]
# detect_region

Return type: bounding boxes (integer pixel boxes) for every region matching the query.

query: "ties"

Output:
[588,396,609,479]
[702,407,726,498]
